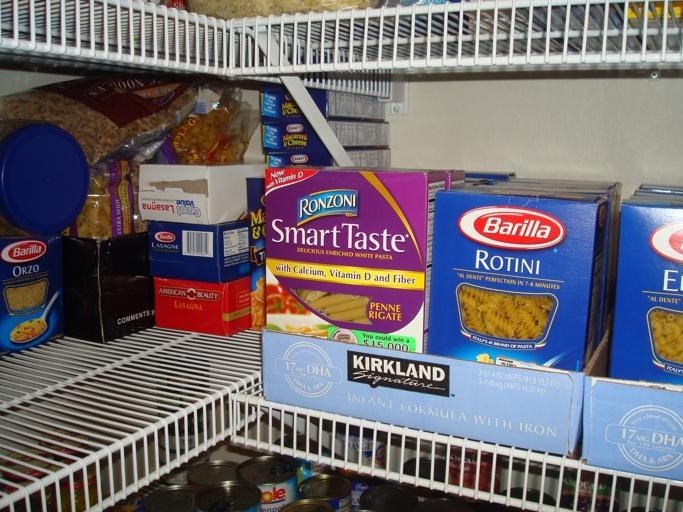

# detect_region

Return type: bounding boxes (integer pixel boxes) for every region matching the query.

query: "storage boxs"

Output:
[261,328,583,459]
[584,317,680,474]
[60,234,156,344]
[139,160,252,335]
[0,234,66,354]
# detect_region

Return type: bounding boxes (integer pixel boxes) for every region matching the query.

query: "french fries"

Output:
[292,288,371,324]
[457,285,552,340]
[650,310,683,363]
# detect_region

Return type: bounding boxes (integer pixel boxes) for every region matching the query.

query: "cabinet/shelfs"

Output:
[224,0,683,512]
[0,0,404,512]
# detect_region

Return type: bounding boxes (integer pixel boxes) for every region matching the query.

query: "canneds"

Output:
[0,441,662,512]
